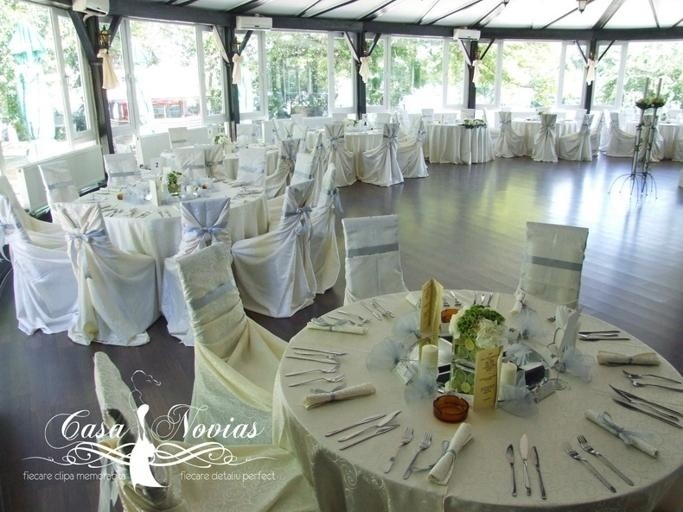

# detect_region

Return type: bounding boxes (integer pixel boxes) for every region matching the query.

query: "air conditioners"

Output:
[72,0,109,17]
[236,16,273,32]
[453,28,481,42]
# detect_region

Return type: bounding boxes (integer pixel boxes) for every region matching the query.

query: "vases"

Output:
[448,339,479,403]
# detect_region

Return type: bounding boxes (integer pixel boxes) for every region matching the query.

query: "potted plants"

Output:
[635,97,652,109]
[652,96,665,108]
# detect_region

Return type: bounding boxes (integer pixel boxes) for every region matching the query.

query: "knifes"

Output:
[360,297,397,323]
[532,445,549,500]
[517,430,532,497]
[506,445,520,496]
[602,382,683,433]
[324,409,405,454]
[578,329,631,341]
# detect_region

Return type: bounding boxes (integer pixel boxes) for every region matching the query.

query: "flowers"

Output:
[441,300,507,350]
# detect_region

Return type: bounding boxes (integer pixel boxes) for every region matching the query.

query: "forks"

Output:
[282,362,343,391]
[438,286,466,308]
[621,370,683,392]
[575,431,638,488]
[325,310,369,326]
[403,429,433,481]
[384,426,415,473]
[562,436,617,494]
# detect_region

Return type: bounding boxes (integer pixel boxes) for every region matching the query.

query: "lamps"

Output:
[576,0,589,12]
[100,24,110,50]
[229,34,240,53]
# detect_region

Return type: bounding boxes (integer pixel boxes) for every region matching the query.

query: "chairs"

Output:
[175,243,289,445]
[517,221,589,306]
[2,87,427,343]
[89,352,321,511]
[427,105,682,187]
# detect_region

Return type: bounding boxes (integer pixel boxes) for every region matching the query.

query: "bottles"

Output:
[420,343,437,399]
[499,359,517,402]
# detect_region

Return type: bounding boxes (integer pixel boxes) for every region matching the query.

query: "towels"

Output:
[427,422,473,486]
[511,291,527,314]
[583,408,658,457]
[595,348,661,366]
[304,382,375,409]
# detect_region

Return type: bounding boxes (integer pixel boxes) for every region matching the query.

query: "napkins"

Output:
[279,290,683,512]
